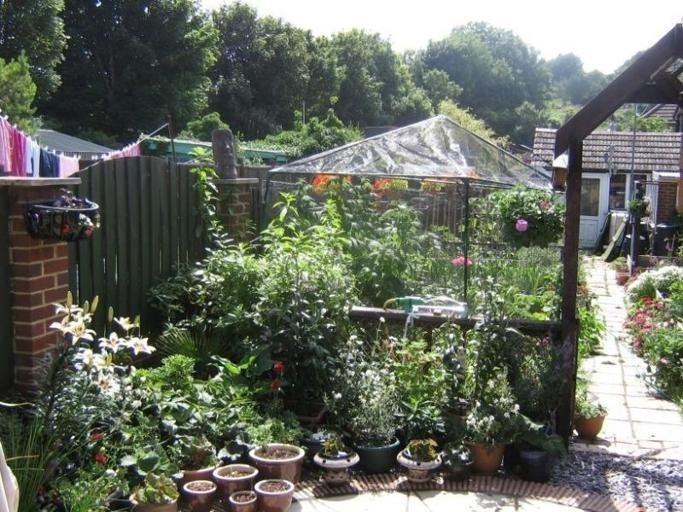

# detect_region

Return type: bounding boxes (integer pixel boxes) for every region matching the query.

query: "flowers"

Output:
[24,189,101,241]
[42,289,156,445]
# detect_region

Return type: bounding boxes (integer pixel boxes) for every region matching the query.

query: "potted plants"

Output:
[211,463,259,501]
[254,478,297,511]
[577,399,607,441]
[182,479,217,510]
[319,375,403,474]
[247,440,308,485]
[129,471,181,510]
[450,396,546,475]
[311,428,365,485]
[226,490,257,510]
[394,437,445,486]
[164,434,223,482]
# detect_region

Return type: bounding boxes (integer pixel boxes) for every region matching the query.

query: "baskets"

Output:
[24,198,99,239]
